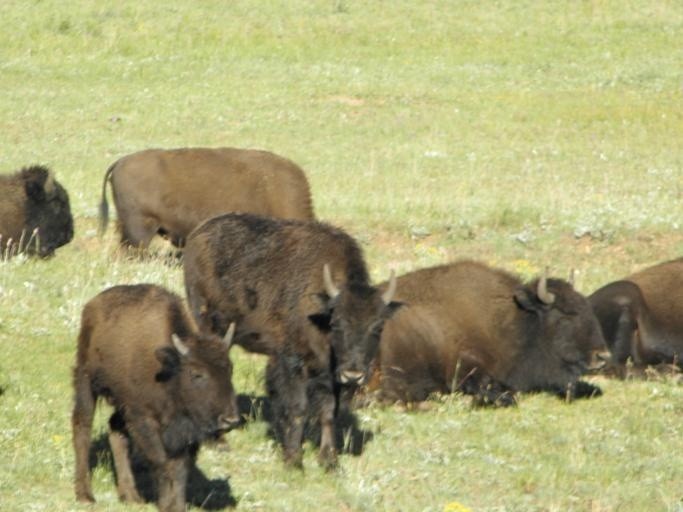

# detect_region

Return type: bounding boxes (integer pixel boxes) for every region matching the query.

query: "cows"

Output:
[0,163,74,260]
[352,261,613,410]
[586,254,682,383]
[96,146,315,262]
[182,210,409,476]
[71,284,245,512]
